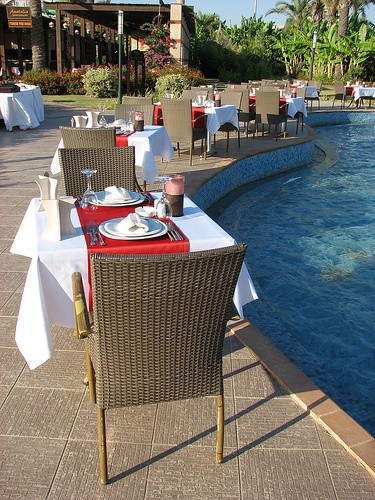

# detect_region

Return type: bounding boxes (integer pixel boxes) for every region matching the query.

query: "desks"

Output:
[0,83,44,131]
[354,87,375,109]
[153,101,240,157]
[304,85,319,110]
[285,98,307,135]
[10,192,259,370]
[49,124,174,192]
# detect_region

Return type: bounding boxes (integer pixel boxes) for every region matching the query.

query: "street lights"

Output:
[310,31,317,81]
[117,9,124,105]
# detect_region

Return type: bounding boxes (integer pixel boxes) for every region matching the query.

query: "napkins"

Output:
[117,213,149,235]
[104,186,132,202]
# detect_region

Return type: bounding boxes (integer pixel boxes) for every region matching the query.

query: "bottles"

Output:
[134,110,145,131]
[158,197,166,218]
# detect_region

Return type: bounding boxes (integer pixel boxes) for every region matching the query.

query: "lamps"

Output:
[48,14,91,36]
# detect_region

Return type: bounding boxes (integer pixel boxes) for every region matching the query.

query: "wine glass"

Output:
[81,169,98,210]
[98,108,107,126]
[126,111,134,131]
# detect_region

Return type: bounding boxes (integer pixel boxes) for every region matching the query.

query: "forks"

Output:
[85,222,105,245]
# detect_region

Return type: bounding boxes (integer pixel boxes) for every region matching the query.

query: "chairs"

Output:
[58,79,356,486]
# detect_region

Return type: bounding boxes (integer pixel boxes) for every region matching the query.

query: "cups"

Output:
[165,175,185,217]
[214,94,221,107]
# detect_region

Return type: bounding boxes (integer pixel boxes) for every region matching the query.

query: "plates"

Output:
[98,216,168,241]
[88,191,145,207]
